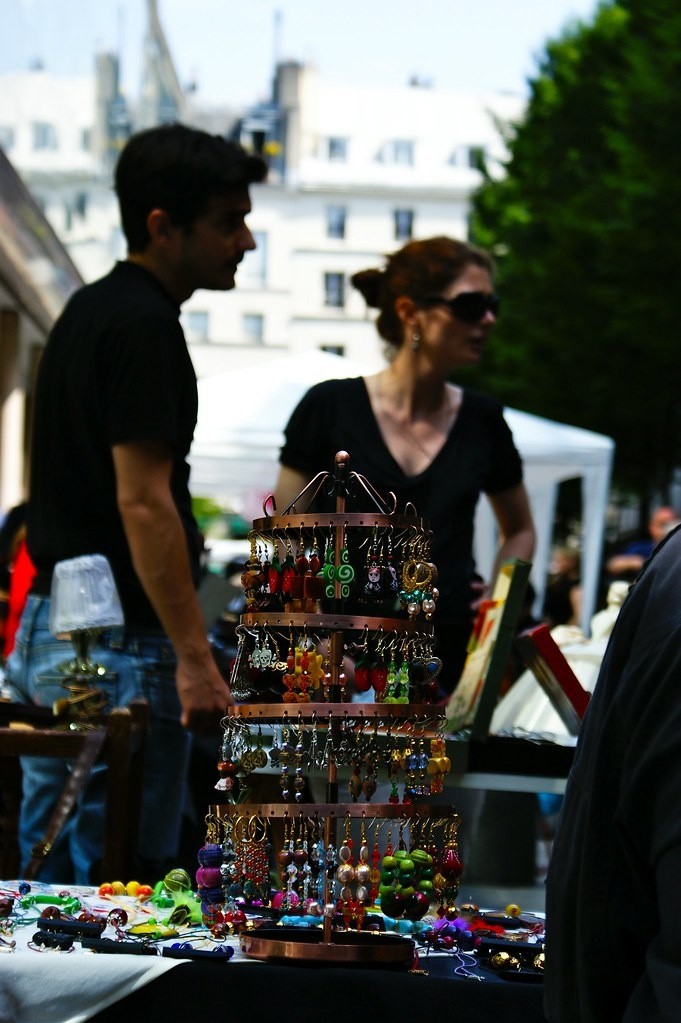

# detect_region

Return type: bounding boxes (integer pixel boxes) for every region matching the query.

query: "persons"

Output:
[1,123,269,886]
[272,235,537,625]
[0,481,681,626]
[542,522,681,1023]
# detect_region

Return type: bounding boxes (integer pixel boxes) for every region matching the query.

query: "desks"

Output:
[0,878,546,1023]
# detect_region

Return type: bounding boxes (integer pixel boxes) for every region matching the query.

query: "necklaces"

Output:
[373,372,451,458]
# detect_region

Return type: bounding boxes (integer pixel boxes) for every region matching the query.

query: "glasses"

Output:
[425,291,501,328]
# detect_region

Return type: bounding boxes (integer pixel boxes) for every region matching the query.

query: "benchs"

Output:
[416,768,568,878]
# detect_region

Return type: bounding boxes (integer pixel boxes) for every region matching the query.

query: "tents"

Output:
[185,340,617,638]
